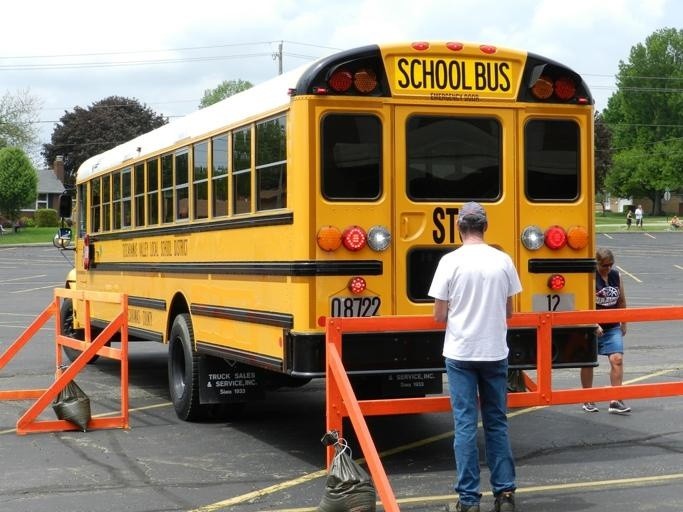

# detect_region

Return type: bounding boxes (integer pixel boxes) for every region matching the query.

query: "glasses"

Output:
[601,263,614,268]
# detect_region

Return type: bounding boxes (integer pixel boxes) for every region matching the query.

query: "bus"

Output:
[51,42,600,421]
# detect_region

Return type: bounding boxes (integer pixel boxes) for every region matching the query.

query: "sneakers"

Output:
[445,499,480,511]
[608,400,631,413]
[583,400,598,411]
[493,490,514,511]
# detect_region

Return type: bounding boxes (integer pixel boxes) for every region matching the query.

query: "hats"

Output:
[457,201,487,225]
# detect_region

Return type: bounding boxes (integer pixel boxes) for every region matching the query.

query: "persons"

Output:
[626,210,633,230]
[581,248,631,413]
[428,202,524,512]
[671,216,680,231]
[635,204,644,228]
